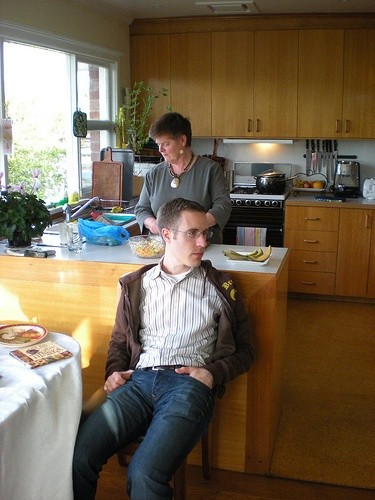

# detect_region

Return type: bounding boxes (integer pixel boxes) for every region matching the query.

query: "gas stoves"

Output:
[227,174,292,201]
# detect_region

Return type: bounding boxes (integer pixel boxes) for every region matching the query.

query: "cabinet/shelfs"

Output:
[337,208,375,303]
[212,14,298,139]
[129,15,212,138]
[284,203,339,300]
[298,12,375,140]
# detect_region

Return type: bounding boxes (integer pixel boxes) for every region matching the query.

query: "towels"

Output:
[236,227,267,246]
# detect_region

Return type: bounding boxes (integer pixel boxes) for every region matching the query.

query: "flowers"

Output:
[0,168,52,242]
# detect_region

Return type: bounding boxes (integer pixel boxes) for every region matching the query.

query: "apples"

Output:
[303,182,311,188]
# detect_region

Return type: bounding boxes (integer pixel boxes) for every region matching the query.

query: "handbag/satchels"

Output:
[77,217,130,246]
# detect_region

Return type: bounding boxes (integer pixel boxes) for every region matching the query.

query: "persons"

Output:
[135,112,232,244]
[73,198,252,500]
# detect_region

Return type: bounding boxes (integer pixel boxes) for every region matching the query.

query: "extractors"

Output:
[223,139,294,146]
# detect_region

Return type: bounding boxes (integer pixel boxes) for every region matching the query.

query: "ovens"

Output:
[221,199,283,248]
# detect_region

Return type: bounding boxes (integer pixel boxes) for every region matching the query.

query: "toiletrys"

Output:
[73,191,78,201]
[64,191,68,204]
[59,194,64,205]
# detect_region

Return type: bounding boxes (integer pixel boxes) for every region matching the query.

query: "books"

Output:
[9,341,72,369]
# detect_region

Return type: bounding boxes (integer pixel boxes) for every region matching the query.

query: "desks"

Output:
[0,329,83,500]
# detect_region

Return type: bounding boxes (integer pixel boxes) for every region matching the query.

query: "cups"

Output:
[68,232,82,252]
[60,223,73,247]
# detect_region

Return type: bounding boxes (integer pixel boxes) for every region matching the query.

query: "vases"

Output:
[8,233,32,248]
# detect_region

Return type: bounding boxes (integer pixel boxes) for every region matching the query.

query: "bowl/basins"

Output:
[127,233,166,259]
[254,169,286,195]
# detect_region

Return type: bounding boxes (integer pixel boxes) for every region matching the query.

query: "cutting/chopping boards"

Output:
[92,148,123,208]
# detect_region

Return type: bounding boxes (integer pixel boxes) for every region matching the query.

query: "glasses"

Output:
[175,229,213,241]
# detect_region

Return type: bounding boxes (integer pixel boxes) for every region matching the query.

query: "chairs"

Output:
[119,387,211,500]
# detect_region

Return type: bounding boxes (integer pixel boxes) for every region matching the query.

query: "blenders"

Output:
[333,160,362,198]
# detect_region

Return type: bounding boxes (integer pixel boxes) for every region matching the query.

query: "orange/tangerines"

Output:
[312,182,324,189]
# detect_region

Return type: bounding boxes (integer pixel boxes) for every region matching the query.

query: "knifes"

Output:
[305,139,338,183]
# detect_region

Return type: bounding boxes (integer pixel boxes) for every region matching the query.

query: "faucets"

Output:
[62,196,100,223]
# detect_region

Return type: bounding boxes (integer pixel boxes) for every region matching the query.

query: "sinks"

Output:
[44,223,78,233]
[72,213,136,226]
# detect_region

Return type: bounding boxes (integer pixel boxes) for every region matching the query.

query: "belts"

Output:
[146,365,178,371]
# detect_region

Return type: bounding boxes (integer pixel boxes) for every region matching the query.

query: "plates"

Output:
[0,324,48,347]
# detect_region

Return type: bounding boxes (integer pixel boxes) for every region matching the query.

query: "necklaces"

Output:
[169,153,195,188]
